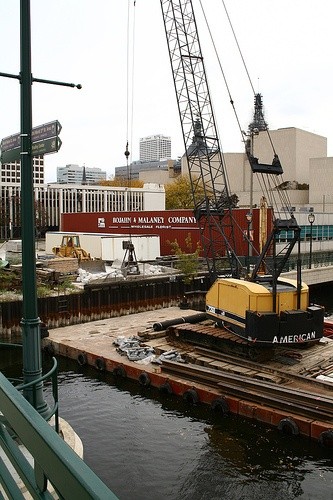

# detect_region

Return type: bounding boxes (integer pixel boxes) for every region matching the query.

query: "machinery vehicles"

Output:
[52,235,106,273]
[159,0,325,364]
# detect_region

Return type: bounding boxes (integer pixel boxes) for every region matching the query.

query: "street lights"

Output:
[245,213,253,271]
[308,214,315,269]
